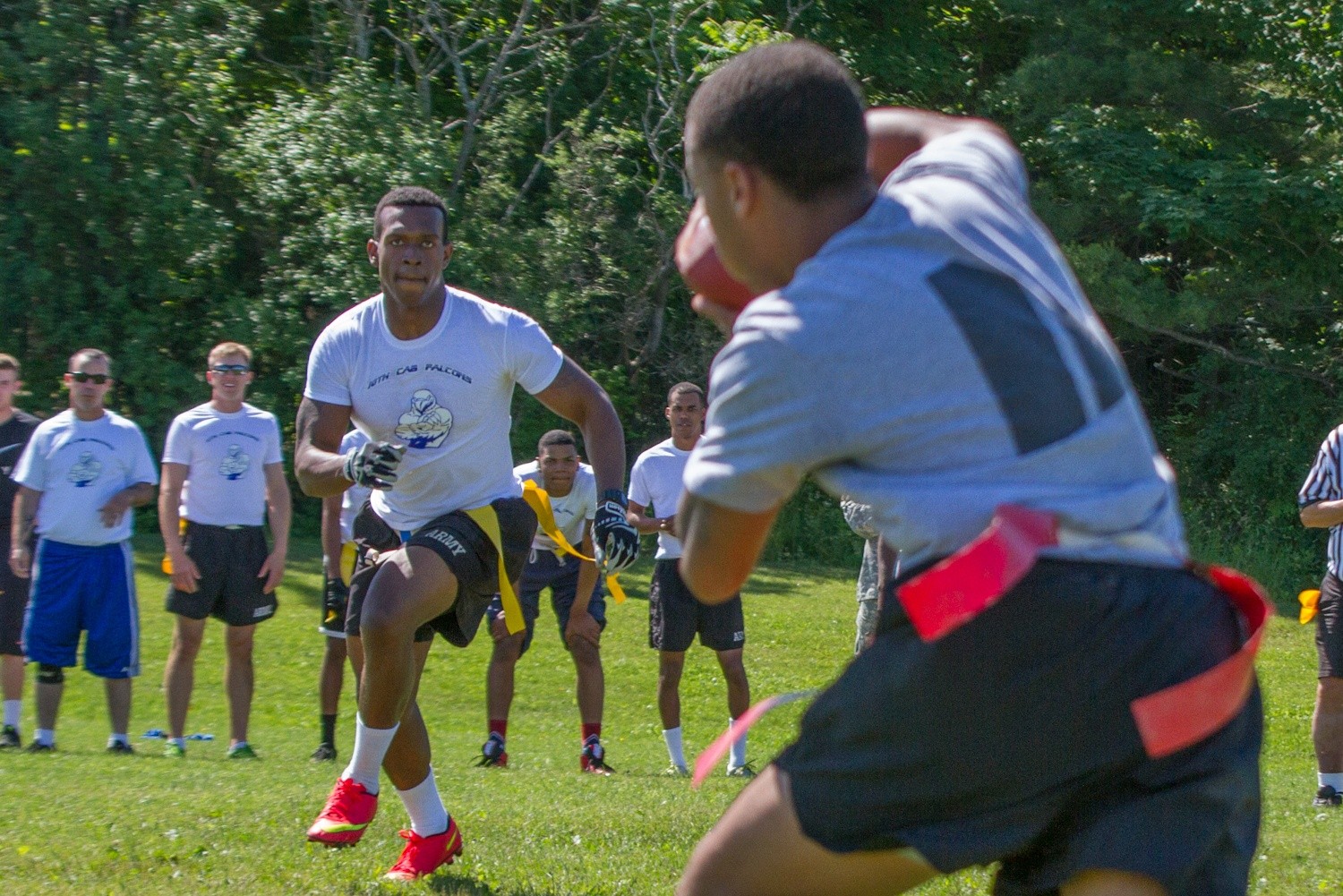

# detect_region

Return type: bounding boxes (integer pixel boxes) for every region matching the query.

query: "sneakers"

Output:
[161,741,185,759]
[227,745,257,760]
[306,780,380,848]
[580,744,615,776]
[382,810,462,883]
[467,738,508,770]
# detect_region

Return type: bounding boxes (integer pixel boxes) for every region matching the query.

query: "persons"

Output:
[149,343,291,761]
[292,187,642,890]
[673,43,1264,896]
[0,352,41,749]
[469,430,618,776]
[627,382,759,778]
[1299,424,1343,806]
[304,428,377,760]
[10,348,158,753]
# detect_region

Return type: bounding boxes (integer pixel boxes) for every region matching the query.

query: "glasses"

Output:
[211,364,249,378]
[71,371,112,386]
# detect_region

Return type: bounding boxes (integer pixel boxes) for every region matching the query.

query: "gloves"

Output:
[592,503,639,573]
[343,441,405,491]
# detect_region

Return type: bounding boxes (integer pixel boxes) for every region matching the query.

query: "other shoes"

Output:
[661,764,690,776]
[22,741,57,755]
[1314,785,1343,816]
[309,745,337,761]
[0,724,21,752]
[103,741,131,756]
[725,759,758,779]
